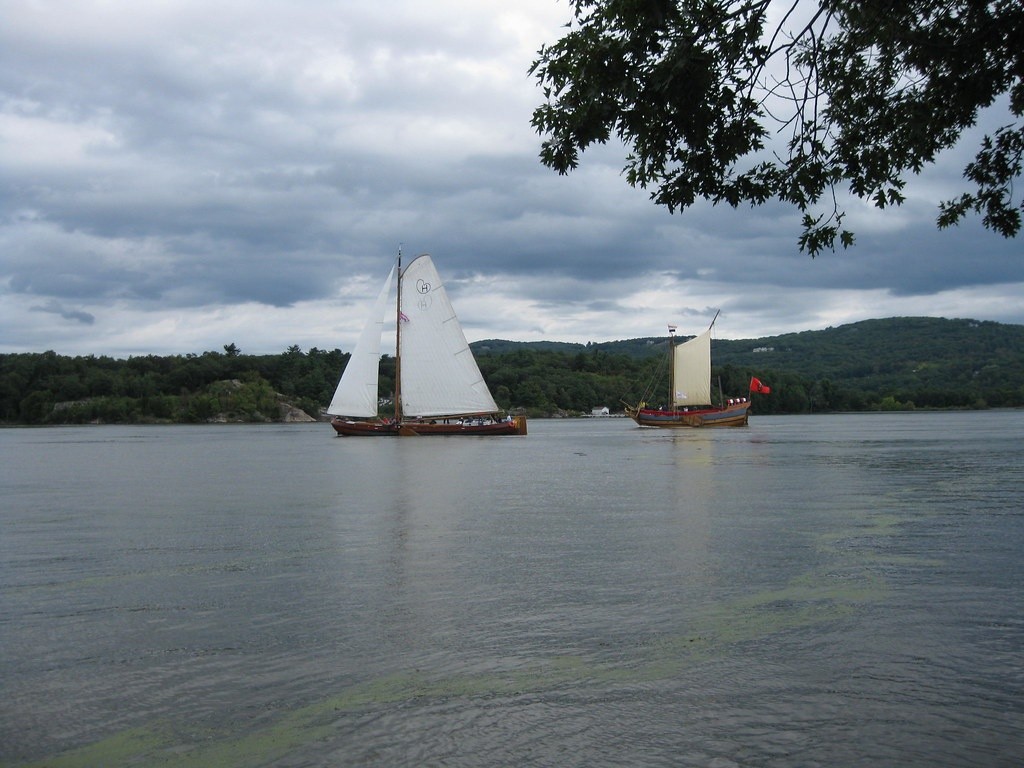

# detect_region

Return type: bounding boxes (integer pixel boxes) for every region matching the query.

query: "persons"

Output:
[458,416,464,425]
[726,397,746,407]
[505,414,511,422]
[468,415,473,425]
[383,417,388,423]
[683,406,689,412]
[444,415,450,424]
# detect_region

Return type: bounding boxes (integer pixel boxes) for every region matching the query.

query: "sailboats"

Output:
[619,308,751,427]
[328,242,528,437]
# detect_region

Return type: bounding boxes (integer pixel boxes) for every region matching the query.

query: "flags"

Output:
[750,376,771,394]
